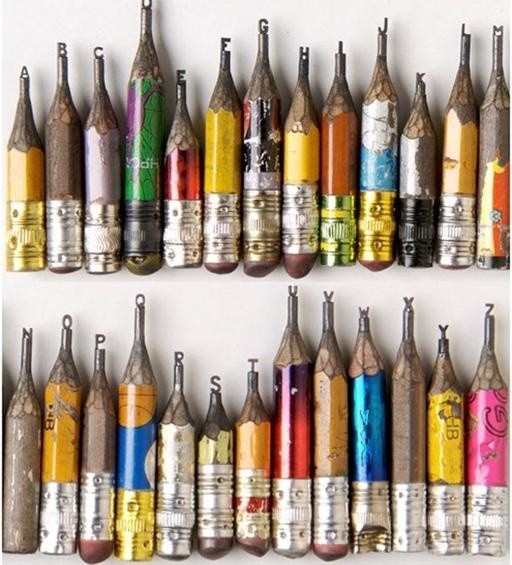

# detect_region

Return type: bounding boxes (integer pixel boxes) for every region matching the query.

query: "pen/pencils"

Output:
[4,0,512,278]
[2,285,512,564]
